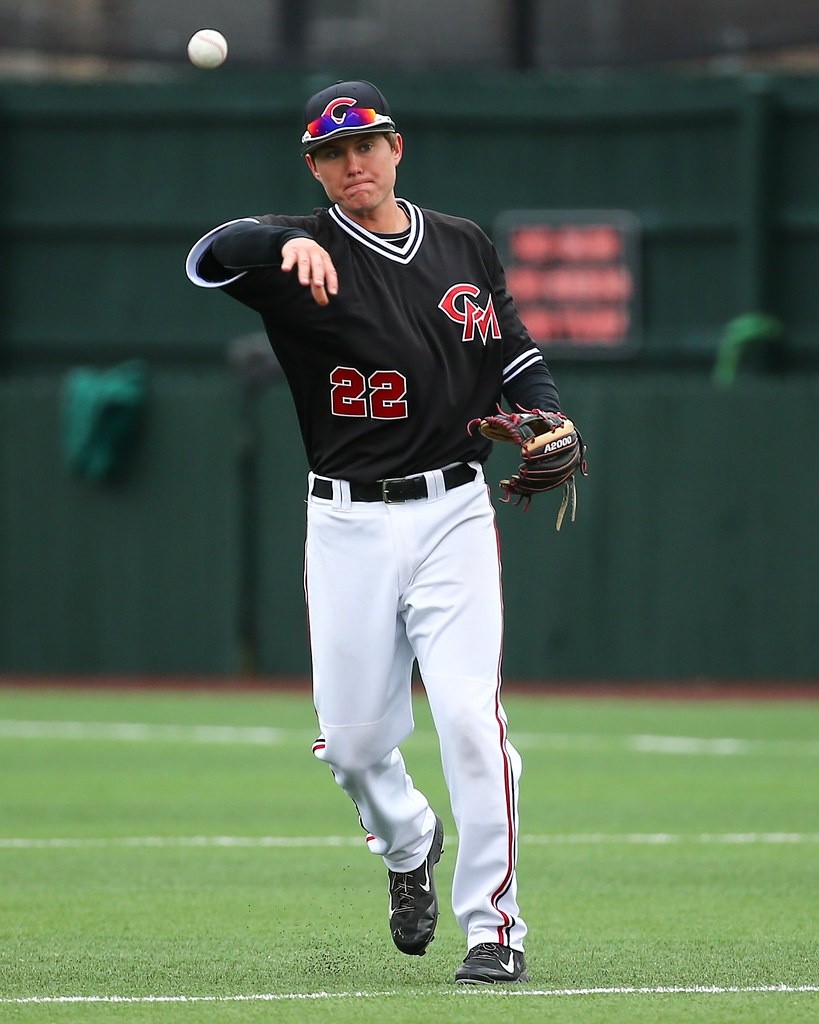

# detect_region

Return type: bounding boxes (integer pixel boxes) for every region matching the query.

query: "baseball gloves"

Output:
[465,406,585,499]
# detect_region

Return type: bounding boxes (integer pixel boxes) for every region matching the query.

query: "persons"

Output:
[185,80,585,986]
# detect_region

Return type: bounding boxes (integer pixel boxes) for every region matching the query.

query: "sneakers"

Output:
[387,816,444,956]
[455,943,530,985]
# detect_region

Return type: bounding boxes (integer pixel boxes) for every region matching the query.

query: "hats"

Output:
[299,79,397,158]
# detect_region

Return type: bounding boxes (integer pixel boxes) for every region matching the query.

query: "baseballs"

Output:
[186,28,227,70]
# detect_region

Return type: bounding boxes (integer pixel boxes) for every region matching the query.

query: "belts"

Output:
[311,464,477,504]
